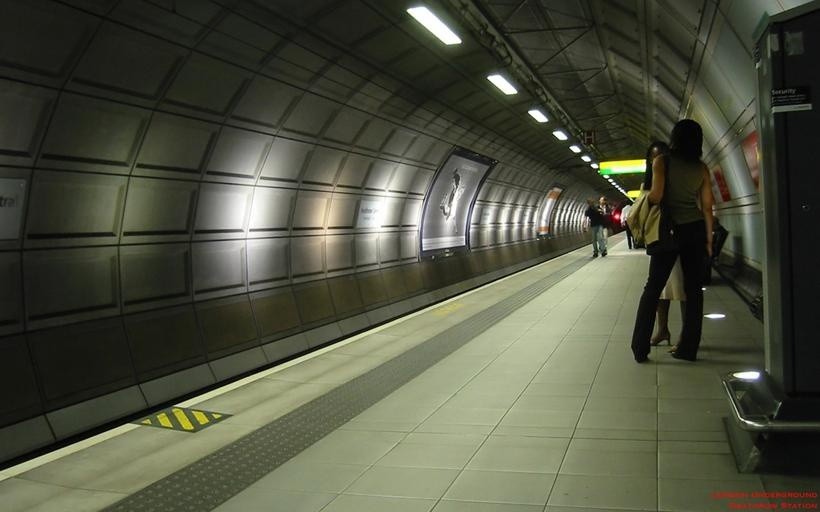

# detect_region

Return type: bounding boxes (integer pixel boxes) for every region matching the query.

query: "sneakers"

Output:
[602,252,607,256]
[593,253,597,257]
[668,344,696,360]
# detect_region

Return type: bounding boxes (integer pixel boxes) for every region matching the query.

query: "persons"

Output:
[596,196,611,249]
[620,118,721,363]
[585,197,607,257]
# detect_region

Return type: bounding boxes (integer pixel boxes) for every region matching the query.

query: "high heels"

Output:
[650,332,671,346]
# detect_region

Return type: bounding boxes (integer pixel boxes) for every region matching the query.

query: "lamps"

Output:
[599,159,647,175]
